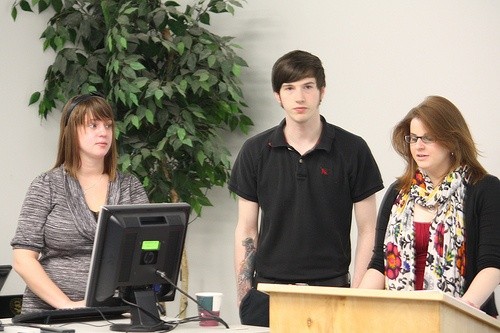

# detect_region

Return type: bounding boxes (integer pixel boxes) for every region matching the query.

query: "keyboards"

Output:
[9,302,133,325]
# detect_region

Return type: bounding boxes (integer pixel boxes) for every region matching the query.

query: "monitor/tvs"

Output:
[85,199,191,333]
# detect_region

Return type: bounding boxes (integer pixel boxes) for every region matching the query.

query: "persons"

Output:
[358,95,500,319]
[10,91,150,310]
[228,50,385,328]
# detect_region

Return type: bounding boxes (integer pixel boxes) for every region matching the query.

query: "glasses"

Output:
[404,132,436,144]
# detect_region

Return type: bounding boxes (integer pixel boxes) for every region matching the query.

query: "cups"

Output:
[195,292,224,327]
